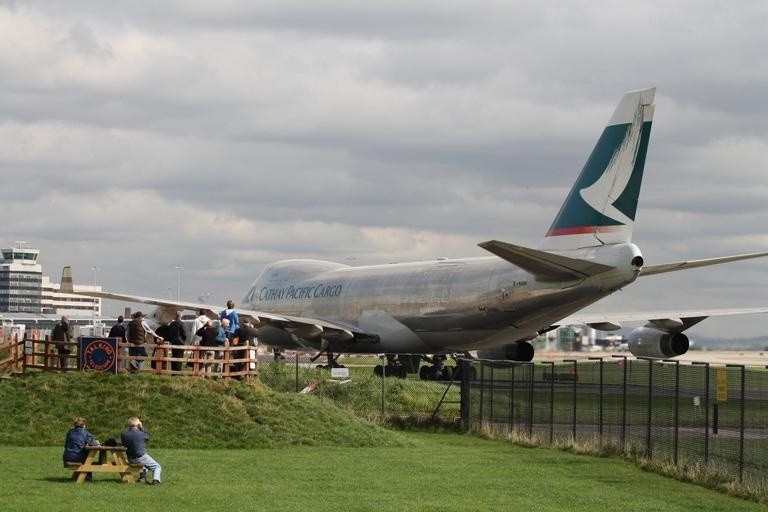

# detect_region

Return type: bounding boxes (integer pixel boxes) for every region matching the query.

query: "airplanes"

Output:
[58,86,766,380]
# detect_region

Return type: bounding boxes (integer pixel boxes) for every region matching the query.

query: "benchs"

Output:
[68,462,143,483]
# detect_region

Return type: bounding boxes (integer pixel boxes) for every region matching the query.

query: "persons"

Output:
[189,308,259,378]
[119,417,163,483]
[106,315,126,356]
[129,312,164,373]
[61,416,97,481]
[220,300,240,346]
[51,314,74,369]
[167,309,189,371]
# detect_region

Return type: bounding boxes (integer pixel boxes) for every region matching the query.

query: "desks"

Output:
[76,445,136,482]
